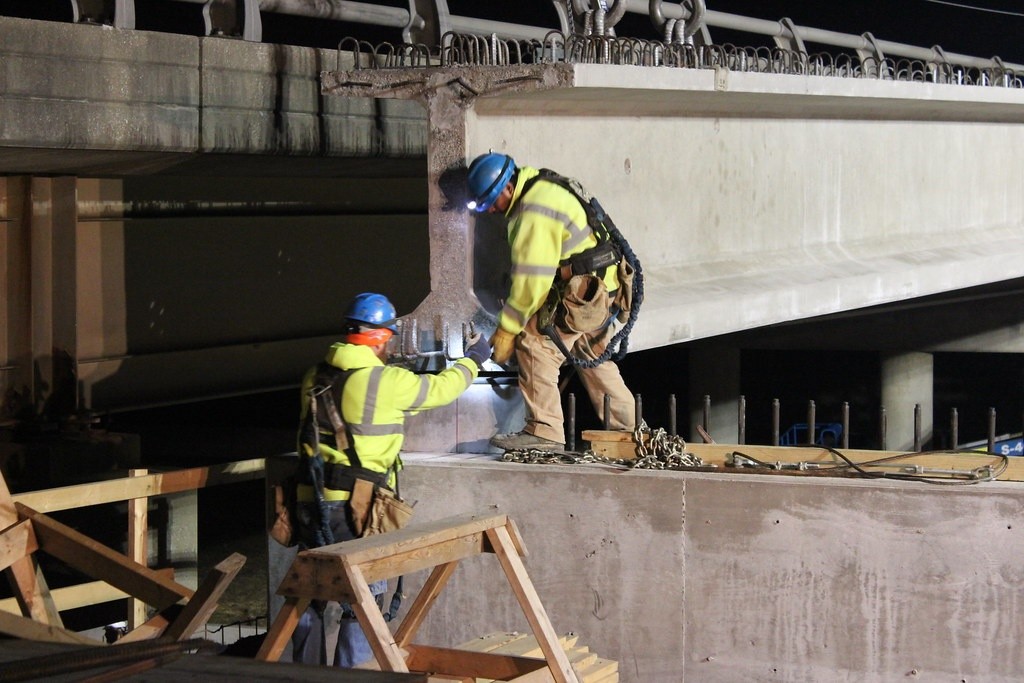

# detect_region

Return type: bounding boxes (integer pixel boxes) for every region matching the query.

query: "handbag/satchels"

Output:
[271,501,294,549]
[361,487,413,538]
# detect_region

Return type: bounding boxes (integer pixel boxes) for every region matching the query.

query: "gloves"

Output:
[486,325,516,364]
[463,331,492,365]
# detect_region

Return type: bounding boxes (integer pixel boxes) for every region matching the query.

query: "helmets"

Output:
[467,152,514,213]
[340,292,399,335]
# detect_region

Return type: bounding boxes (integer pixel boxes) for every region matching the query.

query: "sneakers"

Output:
[489,429,564,452]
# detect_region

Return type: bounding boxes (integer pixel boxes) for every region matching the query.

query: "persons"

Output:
[467,152,646,451]
[291,293,493,666]
